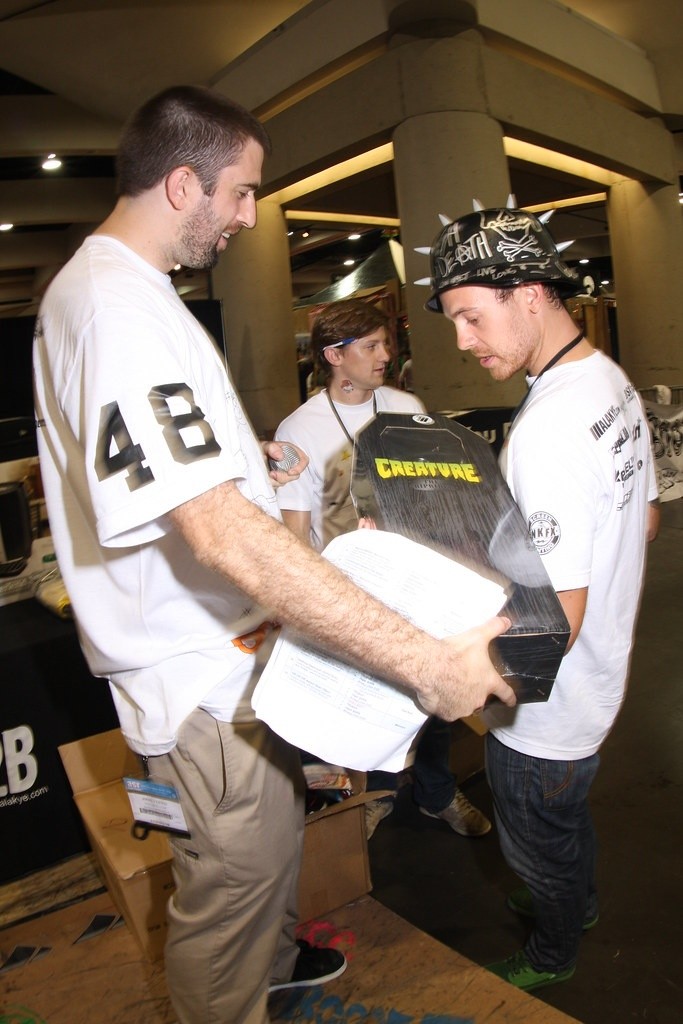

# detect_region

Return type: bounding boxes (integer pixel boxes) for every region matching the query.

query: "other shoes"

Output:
[268,938,347,993]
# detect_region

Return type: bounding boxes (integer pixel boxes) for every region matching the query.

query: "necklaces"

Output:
[326,388,377,448]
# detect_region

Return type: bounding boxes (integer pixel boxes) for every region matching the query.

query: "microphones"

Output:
[266,445,301,472]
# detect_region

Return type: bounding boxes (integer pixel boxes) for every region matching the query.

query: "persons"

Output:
[34,85,517,1024]
[271,297,492,840]
[422,208,658,989]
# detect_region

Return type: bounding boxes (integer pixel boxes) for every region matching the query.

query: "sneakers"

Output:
[465,949,576,998]
[507,886,599,929]
[419,788,491,836]
[365,800,393,840]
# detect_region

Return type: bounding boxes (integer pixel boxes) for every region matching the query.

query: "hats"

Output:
[425,207,579,313]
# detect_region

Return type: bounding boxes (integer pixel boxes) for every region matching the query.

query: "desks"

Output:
[0,535,119,930]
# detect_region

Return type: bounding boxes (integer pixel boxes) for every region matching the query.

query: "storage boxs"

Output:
[349,410,571,706]
[56,727,397,964]
